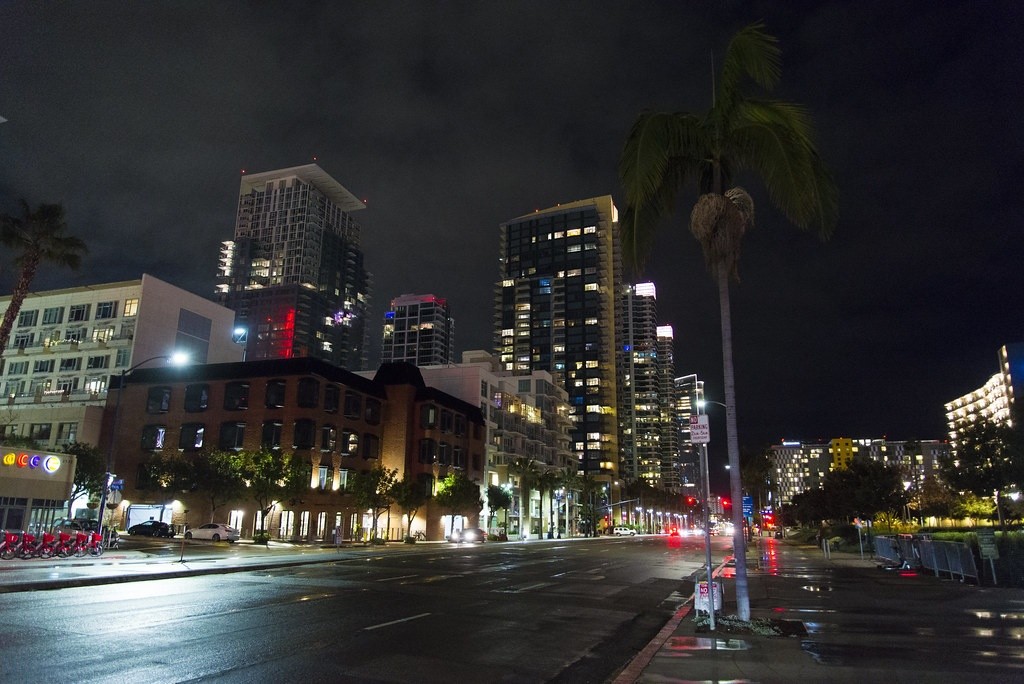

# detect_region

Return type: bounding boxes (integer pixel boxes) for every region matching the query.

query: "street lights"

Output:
[96,353,189,535]
[696,399,750,622]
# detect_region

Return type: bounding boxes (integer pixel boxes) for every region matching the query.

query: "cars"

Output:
[707,521,734,537]
[612,526,636,537]
[452,528,488,544]
[51,517,119,546]
[128,520,176,538]
[184,523,241,544]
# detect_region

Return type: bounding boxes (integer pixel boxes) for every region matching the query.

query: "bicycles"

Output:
[0,526,104,560]
